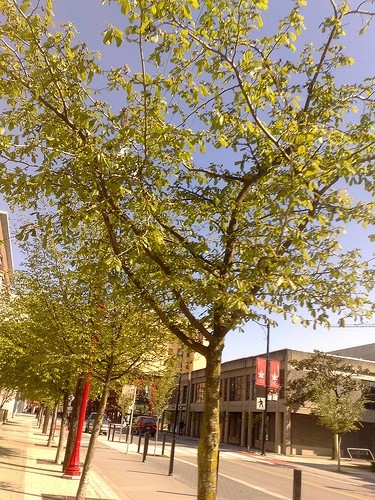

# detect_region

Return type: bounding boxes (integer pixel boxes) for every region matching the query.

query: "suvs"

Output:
[132,417,156,436]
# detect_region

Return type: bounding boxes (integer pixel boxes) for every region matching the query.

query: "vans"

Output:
[87,412,109,436]
